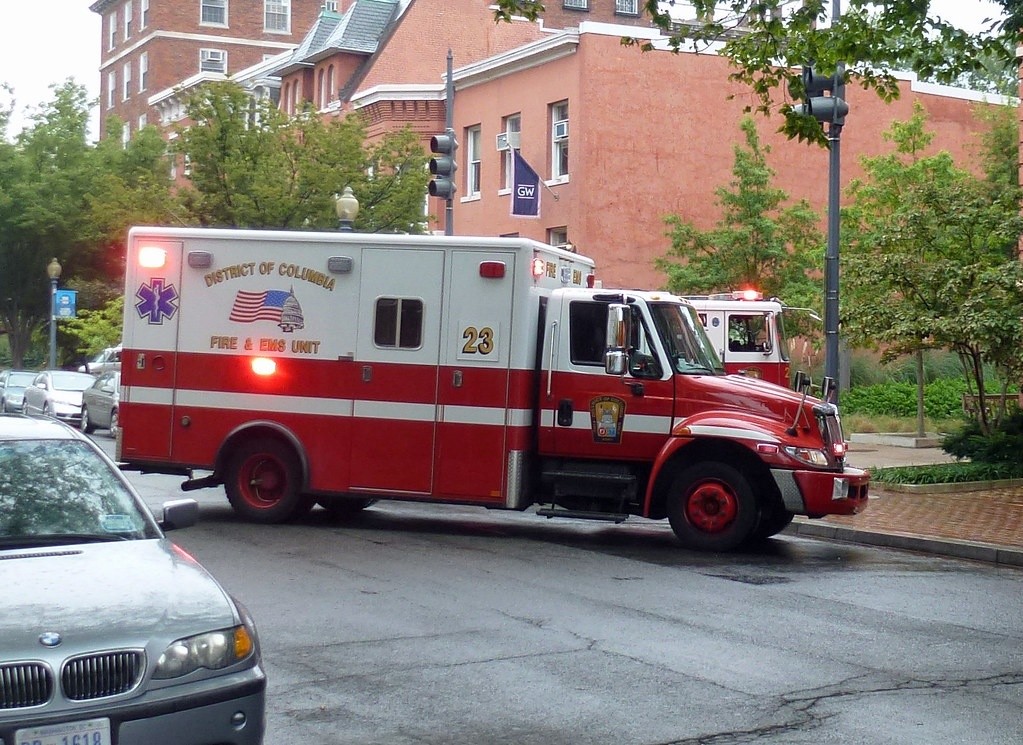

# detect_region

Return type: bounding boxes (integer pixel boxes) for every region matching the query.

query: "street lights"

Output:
[47,257,62,371]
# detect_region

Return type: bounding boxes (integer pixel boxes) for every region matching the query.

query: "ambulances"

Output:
[637,290,823,389]
[114,227,872,554]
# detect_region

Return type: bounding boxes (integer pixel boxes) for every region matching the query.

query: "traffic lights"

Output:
[429,130,455,203]
[789,70,848,132]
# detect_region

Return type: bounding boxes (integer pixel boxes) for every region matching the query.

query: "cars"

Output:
[22,372,95,429]
[0,418,269,744]
[78,348,122,375]
[1,370,41,417]
[80,370,119,438]
